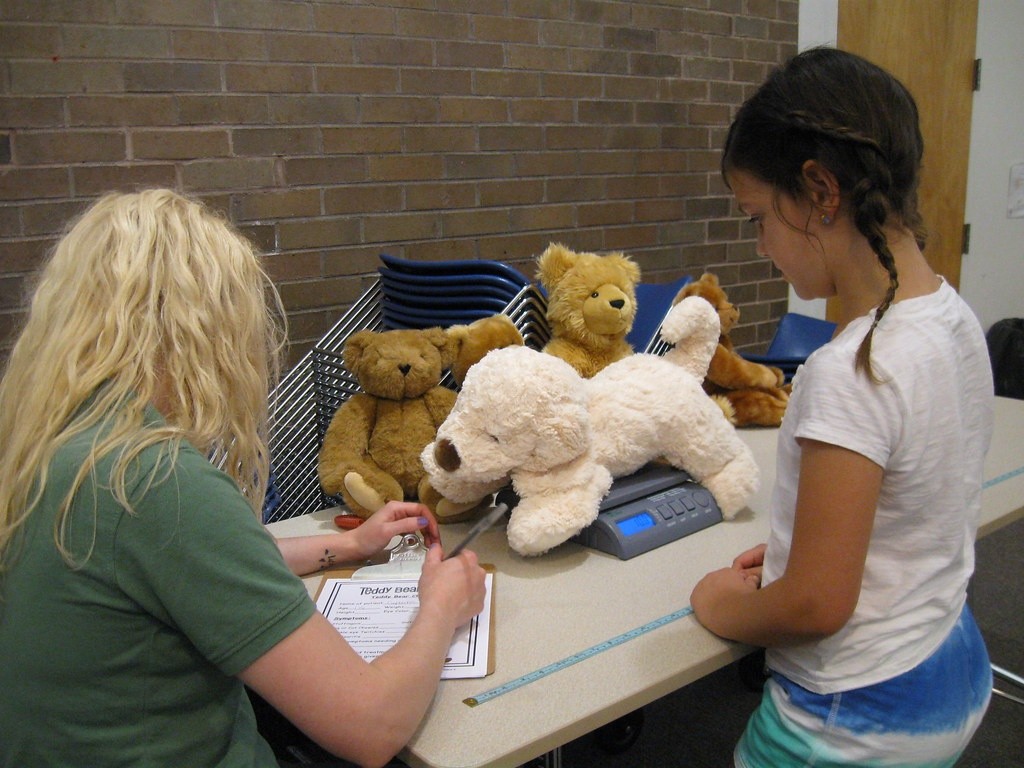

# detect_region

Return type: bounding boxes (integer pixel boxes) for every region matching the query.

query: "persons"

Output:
[691,45,994,768]
[0,190,486,768]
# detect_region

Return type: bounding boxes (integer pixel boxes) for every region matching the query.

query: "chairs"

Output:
[986,317,1024,401]
[739,312,838,386]
[208,253,695,526]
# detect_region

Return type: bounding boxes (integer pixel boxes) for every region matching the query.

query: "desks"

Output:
[262,394,1024,768]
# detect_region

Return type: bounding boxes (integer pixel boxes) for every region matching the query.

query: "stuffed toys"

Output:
[672,273,789,427]
[319,313,524,519]
[535,242,640,378]
[422,297,756,557]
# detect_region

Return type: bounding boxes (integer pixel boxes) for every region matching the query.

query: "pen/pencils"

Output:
[440,501,510,562]
